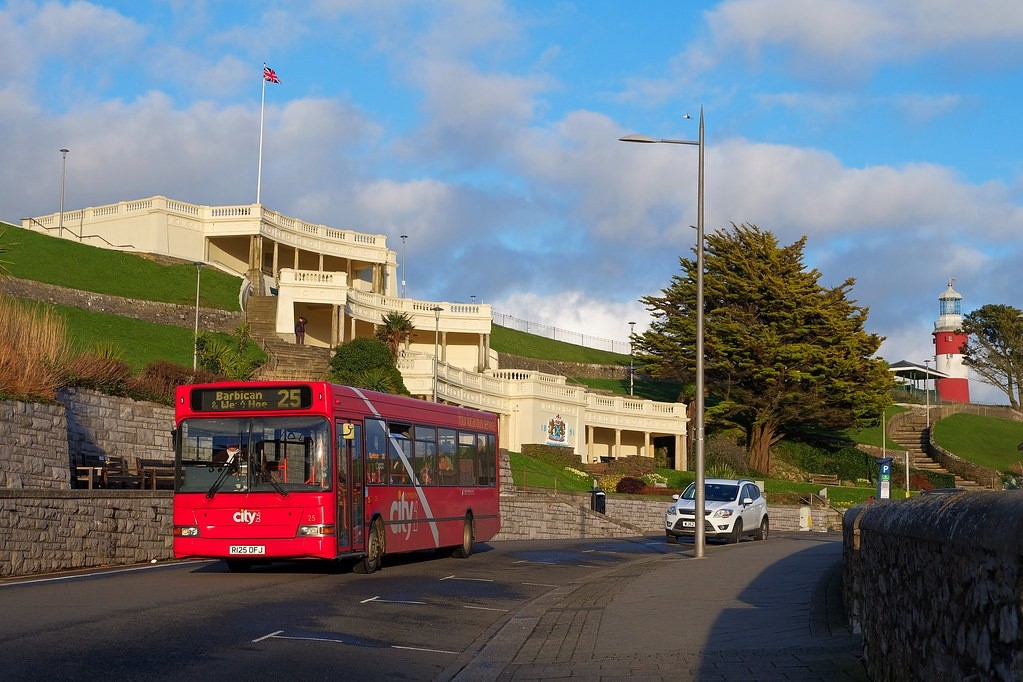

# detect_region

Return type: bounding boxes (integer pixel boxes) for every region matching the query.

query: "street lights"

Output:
[400,235,409,297]
[431,305,444,403]
[628,322,635,395]
[193,262,205,371]
[618,104,703,556]
[59,148,69,238]
[924,360,931,428]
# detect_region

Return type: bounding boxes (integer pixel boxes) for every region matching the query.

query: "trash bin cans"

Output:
[586,490,606,515]
[600,456,615,467]
[799,506,812,531]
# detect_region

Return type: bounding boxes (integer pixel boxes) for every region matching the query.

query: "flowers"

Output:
[644,473,668,485]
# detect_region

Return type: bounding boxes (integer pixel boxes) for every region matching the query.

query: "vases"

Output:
[653,483,667,488]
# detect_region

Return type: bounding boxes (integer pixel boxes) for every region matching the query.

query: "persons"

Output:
[295,317,308,344]
[367,451,393,484]
[472,438,484,453]
[425,445,453,473]
[420,469,430,484]
[213,436,250,476]
[263,453,275,482]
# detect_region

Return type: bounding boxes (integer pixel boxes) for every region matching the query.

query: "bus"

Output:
[171,380,501,574]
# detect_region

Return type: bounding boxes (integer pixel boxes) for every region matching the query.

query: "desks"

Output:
[600,456,615,463]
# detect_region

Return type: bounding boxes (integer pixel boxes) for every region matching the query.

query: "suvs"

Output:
[663,478,770,544]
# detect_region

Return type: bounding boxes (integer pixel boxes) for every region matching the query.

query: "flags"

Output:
[263,62,282,85]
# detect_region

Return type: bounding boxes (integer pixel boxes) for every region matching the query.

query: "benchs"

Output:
[74,451,146,490]
[807,473,841,486]
[135,457,186,490]
